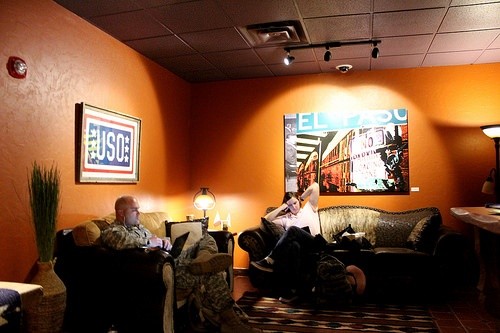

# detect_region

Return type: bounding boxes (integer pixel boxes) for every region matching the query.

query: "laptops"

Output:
[162,232,189,258]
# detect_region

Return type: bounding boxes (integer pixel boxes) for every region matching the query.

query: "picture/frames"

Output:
[76,101,142,183]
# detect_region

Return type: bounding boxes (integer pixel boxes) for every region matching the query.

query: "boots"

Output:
[220,308,261,333]
[189,251,232,275]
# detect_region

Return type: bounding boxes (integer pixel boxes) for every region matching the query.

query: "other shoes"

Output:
[279,289,298,302]
[251,258,274,273]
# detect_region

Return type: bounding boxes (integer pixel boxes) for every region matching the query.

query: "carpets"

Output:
[235,290,440,333]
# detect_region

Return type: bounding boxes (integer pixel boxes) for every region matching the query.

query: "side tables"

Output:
[449,207,500,311]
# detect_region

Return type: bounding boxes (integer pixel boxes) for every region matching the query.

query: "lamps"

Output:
[192,185,217,217]
[371,43,380,58]
[323,47,332,62]
[480,124,500,204]
[284,50,296,65]
[481,168,497,195]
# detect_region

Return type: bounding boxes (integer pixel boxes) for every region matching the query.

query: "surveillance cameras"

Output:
[335,64,353,73]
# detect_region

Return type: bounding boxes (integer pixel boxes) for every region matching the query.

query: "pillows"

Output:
[261,217,286,242]
[169,222,203,244]
[406,213,440,251]
[165,217,208,237]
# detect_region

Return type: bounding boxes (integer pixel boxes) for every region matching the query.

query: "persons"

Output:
[99,196,263,333]
[250,183,322,303]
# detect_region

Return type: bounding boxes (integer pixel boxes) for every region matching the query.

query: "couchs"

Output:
[56,210,235,333]
[237,204,463,303]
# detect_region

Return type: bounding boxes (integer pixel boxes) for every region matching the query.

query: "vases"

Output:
[26,259,66,333]
